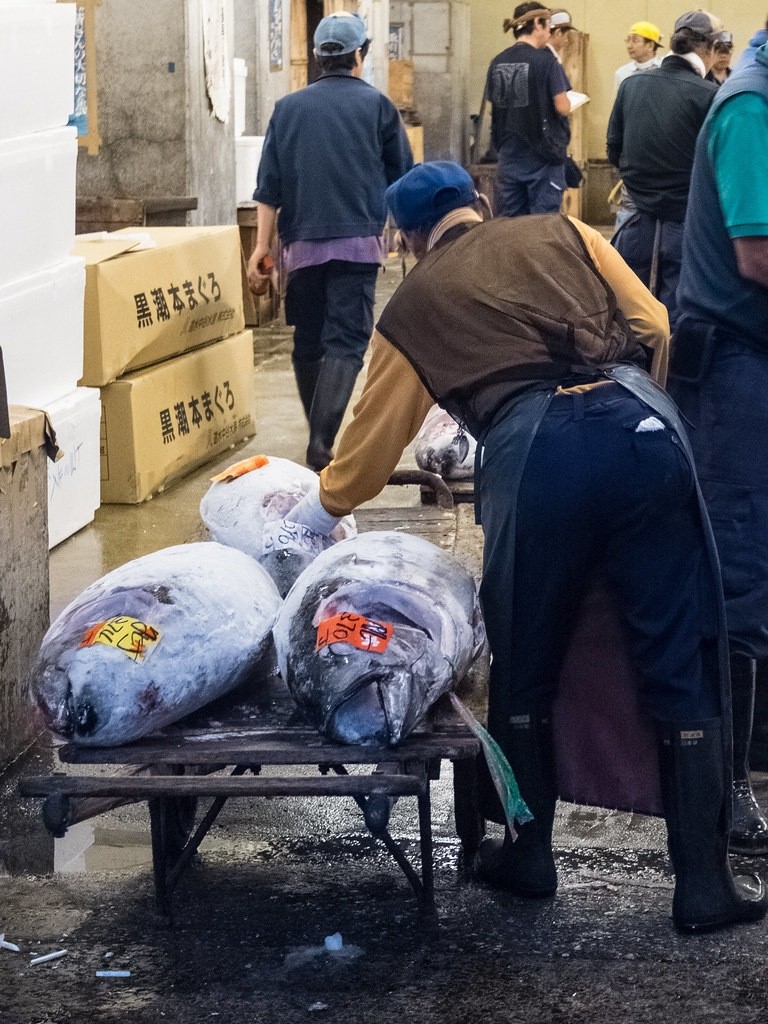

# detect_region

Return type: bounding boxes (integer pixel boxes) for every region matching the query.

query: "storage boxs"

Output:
[0,2,257,550]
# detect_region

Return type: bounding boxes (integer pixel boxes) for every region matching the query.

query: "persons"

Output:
[613,21,664,101]
[606,8,721,337]
[486,1,572,216]
[705,31,734,85]
[283,160,768,933]
[664,16,768,857]
[246,10,414,470]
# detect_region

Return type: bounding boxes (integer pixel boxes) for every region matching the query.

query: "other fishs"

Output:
[272,530,487,751]
[196,456,358,602]
[30,540,281,747]
[416,404,486,483]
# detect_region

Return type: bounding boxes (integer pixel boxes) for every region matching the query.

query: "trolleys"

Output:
[14,464,492,922]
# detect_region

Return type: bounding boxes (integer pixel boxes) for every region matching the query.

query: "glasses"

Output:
[624,38,648,44]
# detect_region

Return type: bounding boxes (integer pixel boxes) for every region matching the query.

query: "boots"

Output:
[475,713,560,895]
[652,718,765,935]
[727,658,768,856]
[292,356,357,469]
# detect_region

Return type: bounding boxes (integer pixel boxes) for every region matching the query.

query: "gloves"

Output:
[282,486,343,537]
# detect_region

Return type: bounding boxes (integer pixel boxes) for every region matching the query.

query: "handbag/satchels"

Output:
[565,157,586,189]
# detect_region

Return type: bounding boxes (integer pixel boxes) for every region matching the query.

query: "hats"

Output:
[629,21,664,48]
[313,8,367,55]
[383,158,475,230]
[674,9,729,41]
[549,8,578,32]
